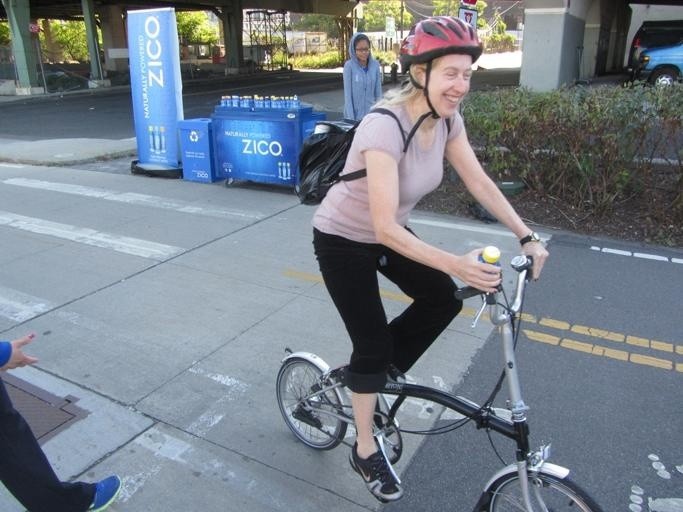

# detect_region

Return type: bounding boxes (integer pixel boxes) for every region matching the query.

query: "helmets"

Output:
[400,16,484,75]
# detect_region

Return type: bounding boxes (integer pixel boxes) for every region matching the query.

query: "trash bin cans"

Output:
[178,118,225,184]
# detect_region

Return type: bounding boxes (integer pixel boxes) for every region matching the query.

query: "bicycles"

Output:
[274,257,603,510]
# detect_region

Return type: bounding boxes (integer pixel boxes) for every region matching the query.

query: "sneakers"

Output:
[350,438,404,504]
[385,362,416,385]
[87,475,120,511]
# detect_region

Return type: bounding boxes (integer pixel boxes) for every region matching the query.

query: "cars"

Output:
[635,39,682,87]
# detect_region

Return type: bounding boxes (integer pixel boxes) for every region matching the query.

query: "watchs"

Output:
[521,231,541,246]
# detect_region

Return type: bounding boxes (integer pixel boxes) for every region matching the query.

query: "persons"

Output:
[464,11,473,23]
[308,13,550,503]
[0,333,123,511]
[340,32,383,124]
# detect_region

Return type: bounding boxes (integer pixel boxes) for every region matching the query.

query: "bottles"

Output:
[277,160,292,182]
[478,246,502,270]
[147,124,166,155]
[220,94,294,109]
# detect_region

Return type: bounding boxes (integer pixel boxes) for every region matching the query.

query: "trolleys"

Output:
[210,105,326,196]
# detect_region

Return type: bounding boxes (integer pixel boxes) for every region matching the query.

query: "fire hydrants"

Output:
[390,62,398,80]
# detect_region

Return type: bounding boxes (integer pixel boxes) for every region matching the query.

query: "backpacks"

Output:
[295,106,451,206]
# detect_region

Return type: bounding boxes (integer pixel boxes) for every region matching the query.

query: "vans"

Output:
[624,20,683,76]
